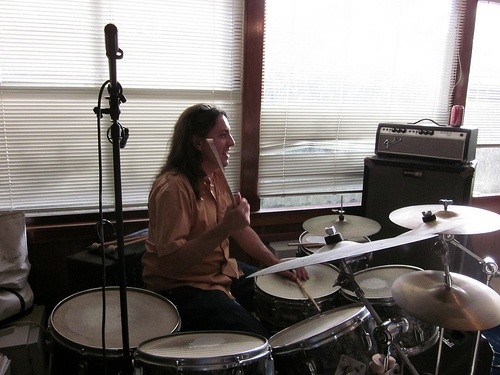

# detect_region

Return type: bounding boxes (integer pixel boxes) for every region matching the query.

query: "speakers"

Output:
[361,154,477,274]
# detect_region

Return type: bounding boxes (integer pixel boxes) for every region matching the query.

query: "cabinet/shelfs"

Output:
[0,304,51,375]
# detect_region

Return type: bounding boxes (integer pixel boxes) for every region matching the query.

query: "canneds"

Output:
[450,105,465,127]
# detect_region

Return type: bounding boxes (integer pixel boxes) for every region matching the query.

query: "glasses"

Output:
[199,104,213,111]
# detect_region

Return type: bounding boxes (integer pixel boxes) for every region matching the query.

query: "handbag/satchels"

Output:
[0,211,34,324]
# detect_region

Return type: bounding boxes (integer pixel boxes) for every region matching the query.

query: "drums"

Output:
[337,265,440,355]
[254,256,343,327]
[130,333,275,375]
[299,229,373,277]
[266,302,398,375]
[46,287,181,375]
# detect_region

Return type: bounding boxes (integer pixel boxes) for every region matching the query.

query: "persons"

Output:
[142,105,309,335]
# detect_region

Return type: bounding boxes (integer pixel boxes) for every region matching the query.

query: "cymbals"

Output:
[390,270,500,331]
[303,215,381,239]
[389,204,500,234]
[395,220,465,236]
[246,233,439,283]
